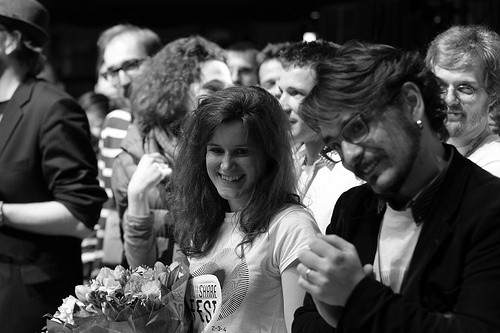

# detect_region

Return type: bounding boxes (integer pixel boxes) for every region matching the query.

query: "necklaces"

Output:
[377,228,387,286]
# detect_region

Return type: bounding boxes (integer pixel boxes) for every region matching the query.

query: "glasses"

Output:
[319,107,370,163]
[106,58,145,81]
[438,86,485,100]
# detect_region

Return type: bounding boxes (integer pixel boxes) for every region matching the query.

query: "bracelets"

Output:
[0,201,3,225]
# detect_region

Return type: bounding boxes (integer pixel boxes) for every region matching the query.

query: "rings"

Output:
[304,268,312,281]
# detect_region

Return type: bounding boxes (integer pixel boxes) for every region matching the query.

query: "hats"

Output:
[0,0,51,38]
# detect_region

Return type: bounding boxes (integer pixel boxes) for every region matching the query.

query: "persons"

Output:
[274,40,367,235]
[112,35,238,267]
[0,3,500,333]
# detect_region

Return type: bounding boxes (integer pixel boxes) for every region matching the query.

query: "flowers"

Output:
[40,261,196,333]
